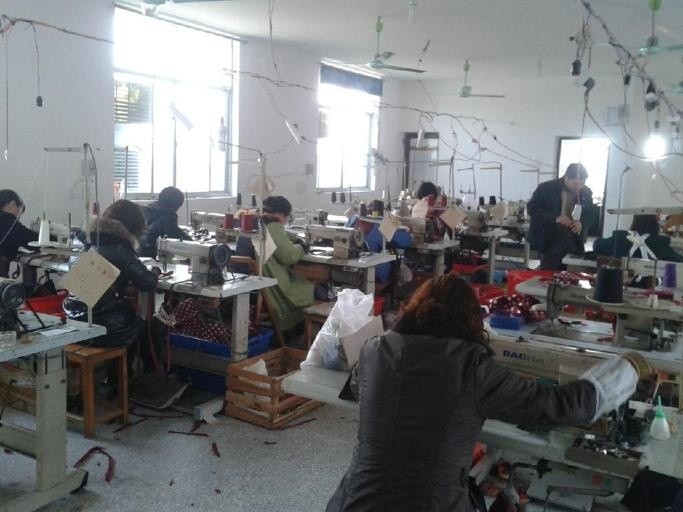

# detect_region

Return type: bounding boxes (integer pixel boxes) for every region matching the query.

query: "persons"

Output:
[137,185,193,263]
[0,188,57,280]
[414,181,437,205]
[75,199,161,390]
[343,199,413,283]
[318,270,662,510]
[235,195,315,350]
[593,215,682,263]
[525,162,601,258]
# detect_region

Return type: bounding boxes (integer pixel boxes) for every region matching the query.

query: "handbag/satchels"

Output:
[338,313,386,371]
[25,280,69,320]
[619,465,683,512]
[315,280,336,302]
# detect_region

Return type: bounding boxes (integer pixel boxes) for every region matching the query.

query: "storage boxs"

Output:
[170,326,329,430]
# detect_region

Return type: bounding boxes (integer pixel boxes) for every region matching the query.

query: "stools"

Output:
[305,301,336,349]
[66,344,128,440]
